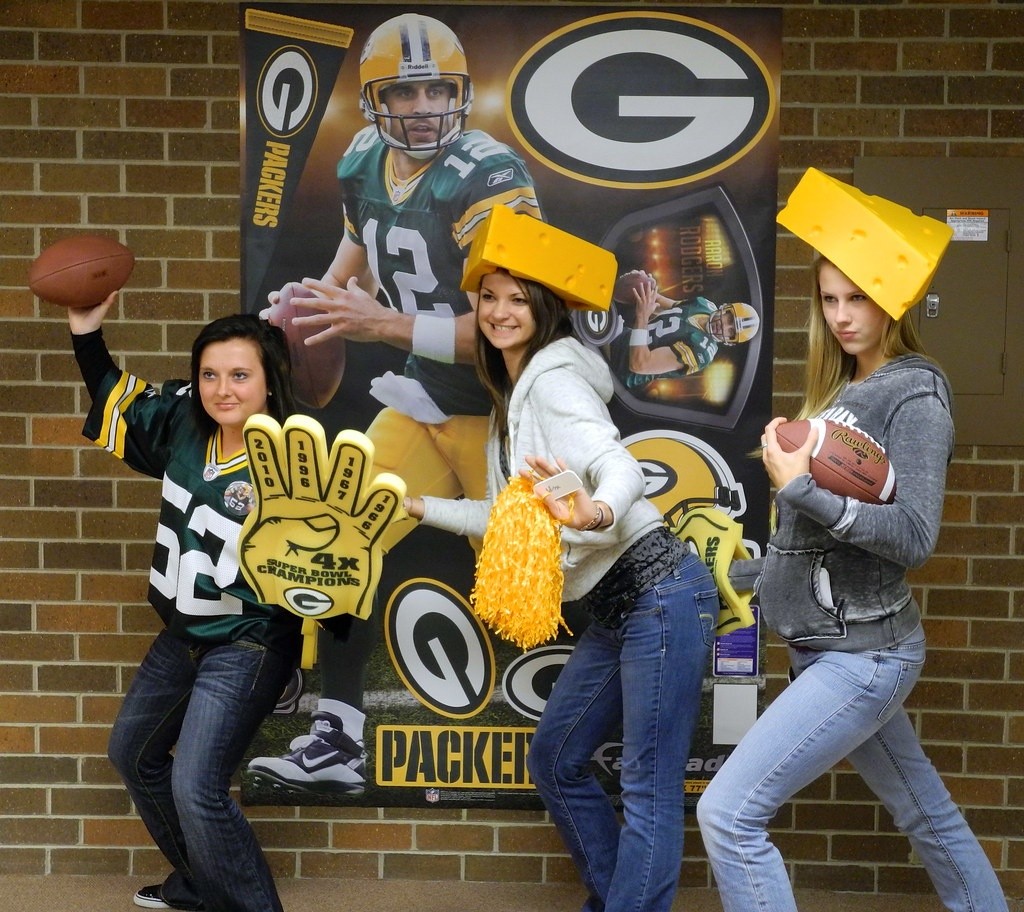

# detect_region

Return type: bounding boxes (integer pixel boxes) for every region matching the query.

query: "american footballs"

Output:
[775,418,898,506]
[268,280,347,410]
[27,234,138,309]
[613,273,658,304]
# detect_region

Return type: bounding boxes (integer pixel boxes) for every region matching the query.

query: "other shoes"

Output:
[133,882,171,908]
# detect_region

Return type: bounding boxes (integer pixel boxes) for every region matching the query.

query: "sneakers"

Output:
[247,711,369,801]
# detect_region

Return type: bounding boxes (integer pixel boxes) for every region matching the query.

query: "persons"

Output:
[64,287,408,912]
[249,7,550,797]
[692,238,1014,910]
[600,267,761,388]
[404,258,726,910]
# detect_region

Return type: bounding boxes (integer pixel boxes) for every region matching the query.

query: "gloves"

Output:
[236,414,407,622]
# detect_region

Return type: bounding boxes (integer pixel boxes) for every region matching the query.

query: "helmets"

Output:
[706,301,760,348]
[359,13,475,159]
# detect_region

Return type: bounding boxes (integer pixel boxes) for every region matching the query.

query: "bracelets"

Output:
[576,505,604,533]
